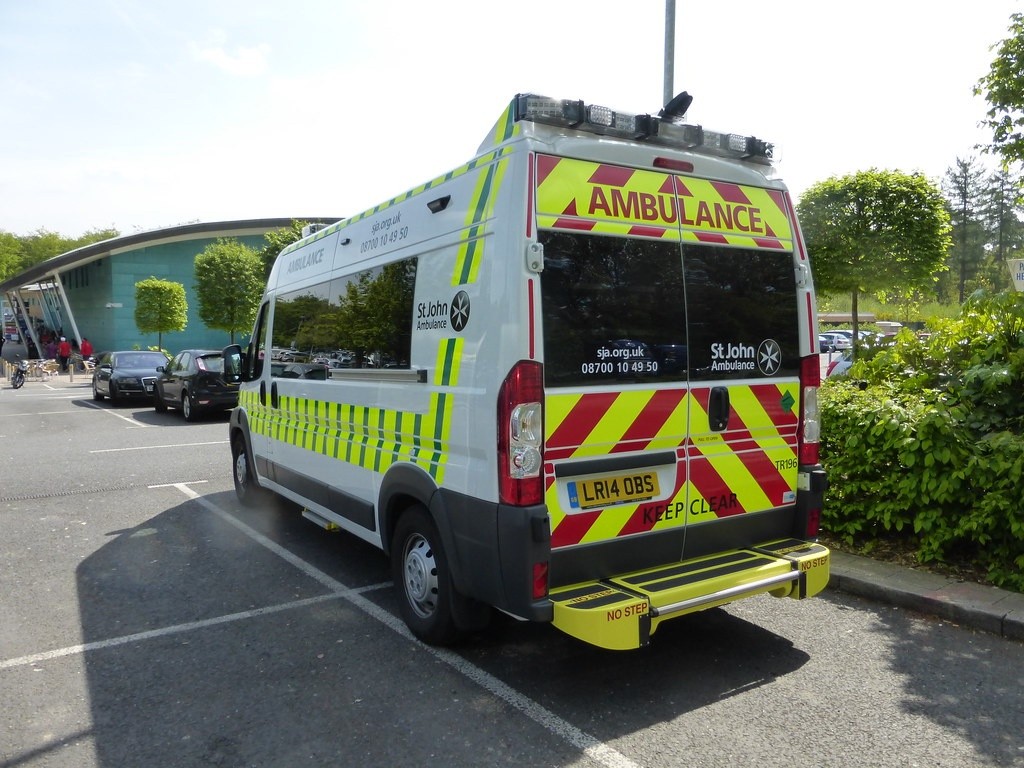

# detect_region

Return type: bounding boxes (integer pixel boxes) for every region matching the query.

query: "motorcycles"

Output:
[9,353,32,389]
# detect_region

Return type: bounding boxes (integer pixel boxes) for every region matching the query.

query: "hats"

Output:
[61,337,66,341]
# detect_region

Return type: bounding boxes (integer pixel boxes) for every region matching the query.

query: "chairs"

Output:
[83,361,96,378]
[24,358,60,383]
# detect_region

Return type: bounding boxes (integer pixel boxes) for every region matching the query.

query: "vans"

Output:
[825,330,864,347]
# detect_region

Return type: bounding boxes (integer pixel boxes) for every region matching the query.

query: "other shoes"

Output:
[64,369,69,373]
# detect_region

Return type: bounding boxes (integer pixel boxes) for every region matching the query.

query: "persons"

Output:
[80,338,93,370]
[45,340,57,359]
[58,337,72,371]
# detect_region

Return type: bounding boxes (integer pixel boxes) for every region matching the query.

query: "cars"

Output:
[91,351,172,403]
[276,350,375,367]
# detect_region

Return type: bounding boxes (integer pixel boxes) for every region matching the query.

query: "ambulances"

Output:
[229,91,831,670]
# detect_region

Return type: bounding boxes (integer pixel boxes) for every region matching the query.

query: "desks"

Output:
[30,359,45,371]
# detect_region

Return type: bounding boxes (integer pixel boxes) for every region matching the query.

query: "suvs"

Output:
[819,334,853,353]
[153,350,243,423]
